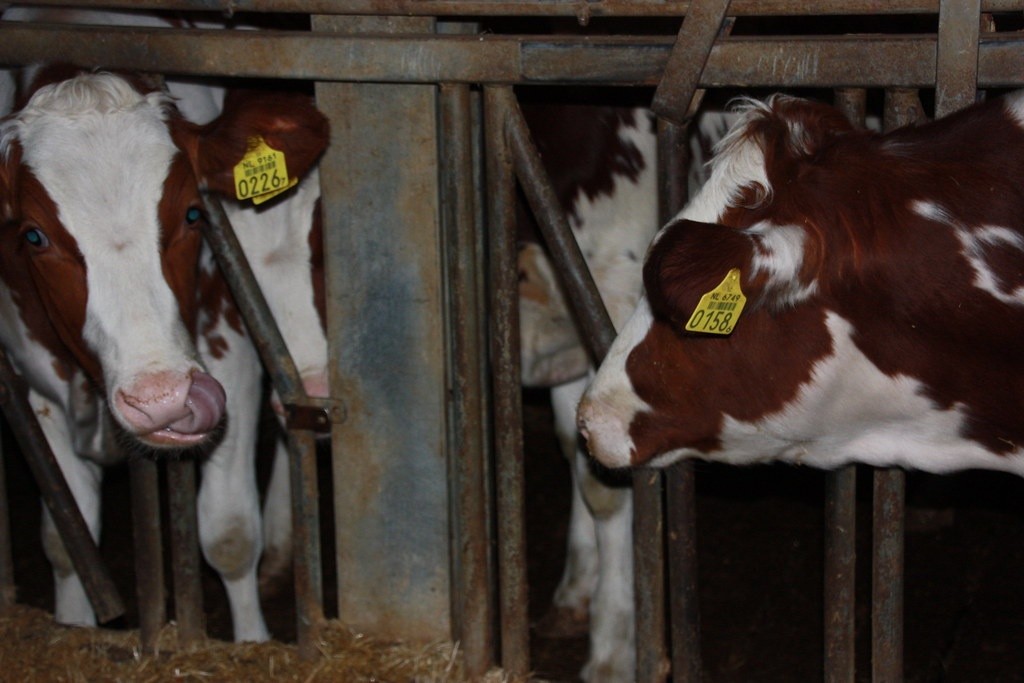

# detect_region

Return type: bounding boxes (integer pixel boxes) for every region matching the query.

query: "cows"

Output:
[0,0,1024,683]
